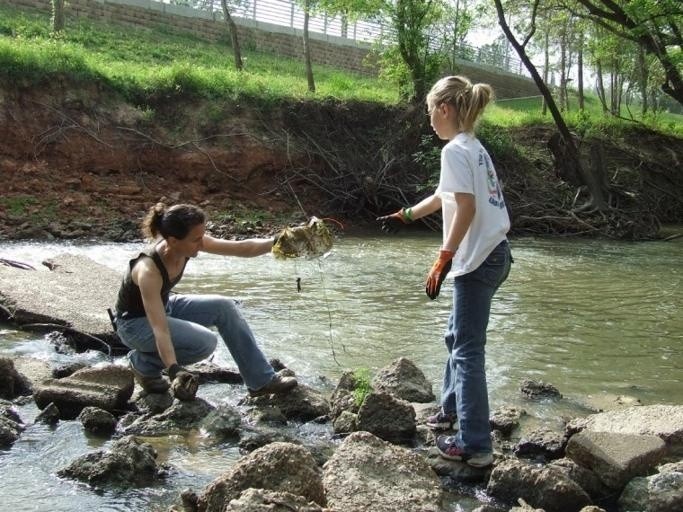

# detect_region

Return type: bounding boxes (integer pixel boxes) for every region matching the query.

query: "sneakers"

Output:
[129,356,170,392]
[435,433,494,467]
[426,412,459,430]
[248,375,297,396]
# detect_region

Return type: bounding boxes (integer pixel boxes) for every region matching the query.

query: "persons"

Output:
[112,203,306,402]
[374,76,516,469]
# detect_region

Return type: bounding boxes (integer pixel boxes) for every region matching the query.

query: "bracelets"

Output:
[404,208,414,223]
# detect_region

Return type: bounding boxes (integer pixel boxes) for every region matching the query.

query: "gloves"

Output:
[167,363,198,401]
[375,206,411,236]
[424,249,457,300]
